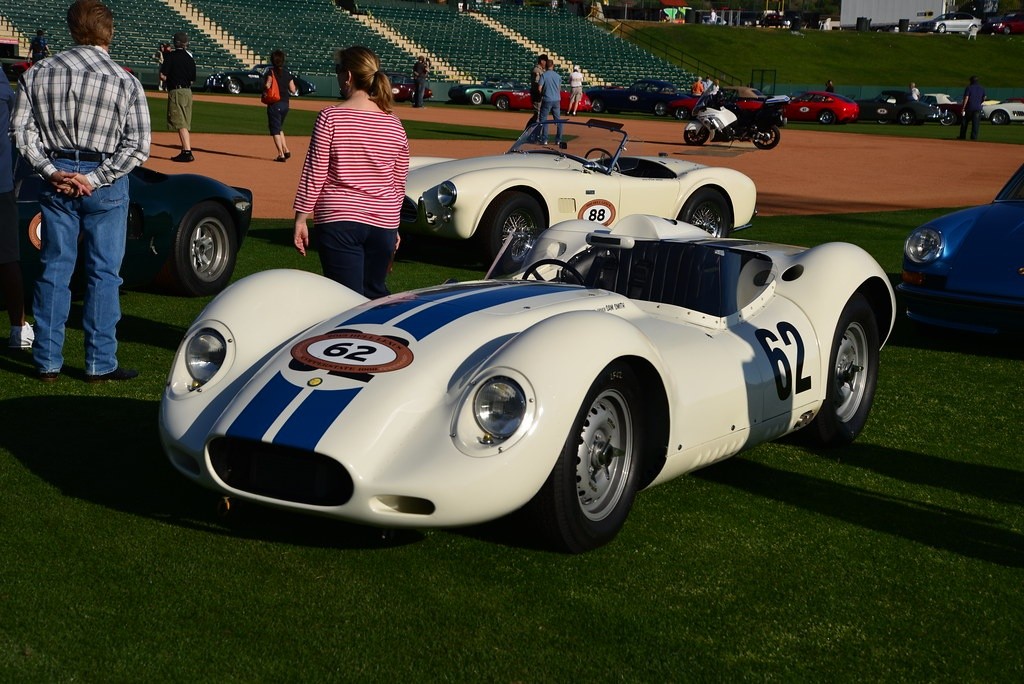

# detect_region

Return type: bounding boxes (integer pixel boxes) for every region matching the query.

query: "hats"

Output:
[574,65,580,71]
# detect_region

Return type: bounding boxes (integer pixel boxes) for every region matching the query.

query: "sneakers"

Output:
[9,322,35,348]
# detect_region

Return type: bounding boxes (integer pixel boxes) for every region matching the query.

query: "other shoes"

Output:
[556,142,560,145]
[540,141,547,145]
[566,112,569,114]
[957,136,961,139]
[171,154,194,162]
[572,112,576,115]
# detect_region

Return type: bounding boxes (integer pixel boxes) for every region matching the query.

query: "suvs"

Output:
[760,14,782,28]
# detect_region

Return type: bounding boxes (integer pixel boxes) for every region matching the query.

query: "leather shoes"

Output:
[91,366,139,380]
[42,373,59,381]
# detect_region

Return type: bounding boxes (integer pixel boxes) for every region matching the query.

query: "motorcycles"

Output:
[685,82,782,150]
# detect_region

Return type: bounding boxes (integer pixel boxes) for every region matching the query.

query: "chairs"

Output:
[0,0,701,91]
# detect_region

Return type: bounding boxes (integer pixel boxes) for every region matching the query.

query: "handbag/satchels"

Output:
[261,70,280,104]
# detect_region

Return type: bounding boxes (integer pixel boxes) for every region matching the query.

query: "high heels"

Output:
[285,153,290,158]
[273,155,285,162]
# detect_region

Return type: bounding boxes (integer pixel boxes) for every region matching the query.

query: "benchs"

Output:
[585,241,741,317]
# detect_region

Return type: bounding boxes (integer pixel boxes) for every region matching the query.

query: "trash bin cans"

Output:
[867,18,872,31]
[685,9,695,23]
[857,17,867,30]
[899,19,909,32]
[695,12,703,24]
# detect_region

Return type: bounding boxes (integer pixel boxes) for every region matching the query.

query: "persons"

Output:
[825,80,834,100]
[293,46,409,299]
[956,76,986,139]
[710,9,716,21]
[413,55,430,109]
[692,75,719,95]
[909,82,920,100]
[159,32,194,162]
[152,43,171,92]
[525,54,582,145]
[259,50,296,162]
[0,0,152,382]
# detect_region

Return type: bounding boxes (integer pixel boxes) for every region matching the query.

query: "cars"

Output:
[450,77,693,116]
[670,87,860,126]
[399,119,757,275]
[205,64,316,98]
[876,88,1024,127]
[14,167,252,297]
[894,162,1024,340]
[340,71,434,104]
[927,11,1024,35]
[158,214,893,555]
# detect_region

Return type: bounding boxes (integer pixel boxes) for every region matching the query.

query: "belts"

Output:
[47,151,113,161]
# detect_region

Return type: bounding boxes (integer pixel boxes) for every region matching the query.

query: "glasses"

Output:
[336,64,347,74]
